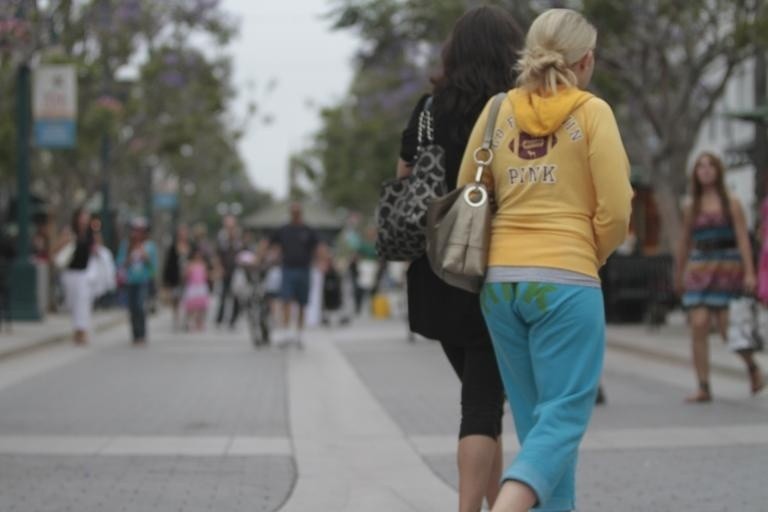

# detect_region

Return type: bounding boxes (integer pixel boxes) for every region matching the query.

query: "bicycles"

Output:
[235,251,275,343]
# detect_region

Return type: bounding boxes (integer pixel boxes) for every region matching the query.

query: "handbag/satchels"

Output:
[728,294,762,352]
[374,145,447,260]
[426,182,491,294]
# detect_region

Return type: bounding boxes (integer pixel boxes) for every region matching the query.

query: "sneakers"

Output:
[750,369,764,390]
[685,391,711,403]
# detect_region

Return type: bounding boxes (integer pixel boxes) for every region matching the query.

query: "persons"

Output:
[453,6,637,511]
[394,3,527,512]
[751,191,768,353]
[32,192,388,349]
[671,149,766,404]
